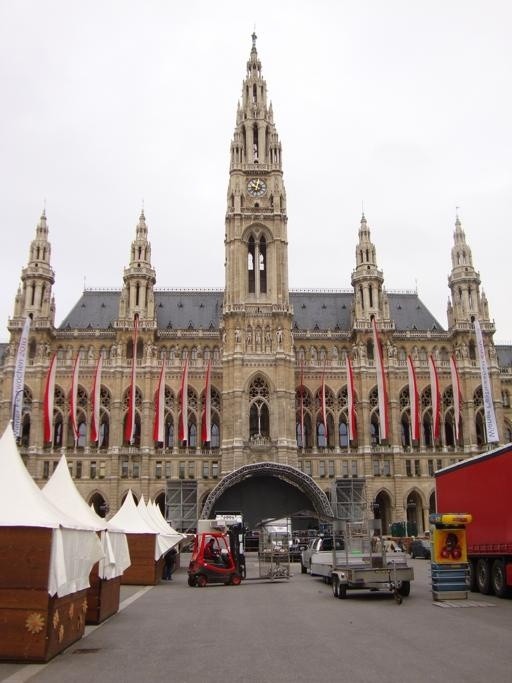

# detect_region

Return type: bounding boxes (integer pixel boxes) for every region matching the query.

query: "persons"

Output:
[161,548,177,581]
[205,538,220,560]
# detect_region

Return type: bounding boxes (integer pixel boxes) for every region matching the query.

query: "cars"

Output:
[245,528,432,598]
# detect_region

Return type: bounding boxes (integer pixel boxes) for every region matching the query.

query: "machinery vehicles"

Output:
[187,523,249,587]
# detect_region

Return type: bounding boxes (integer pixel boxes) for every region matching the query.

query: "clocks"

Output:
[244,177,266,196]
